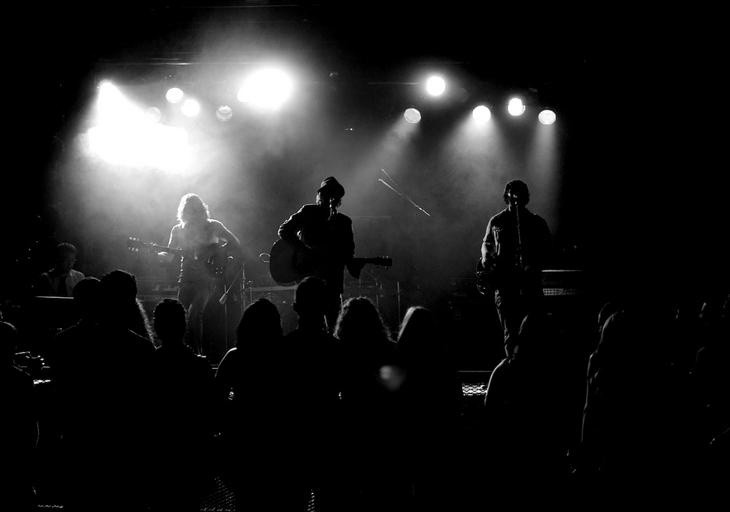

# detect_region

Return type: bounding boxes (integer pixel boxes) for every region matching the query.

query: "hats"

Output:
[316,176,345,198]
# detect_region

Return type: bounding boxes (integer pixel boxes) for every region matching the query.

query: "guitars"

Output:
[127,236,247,276]
[269,238,391,287]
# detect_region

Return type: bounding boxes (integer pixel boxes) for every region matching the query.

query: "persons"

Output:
[39,241,86,293]
[134,192,241,354]
[0,268,730,512]
[479,177,572,359]
[276,174,356,333]
